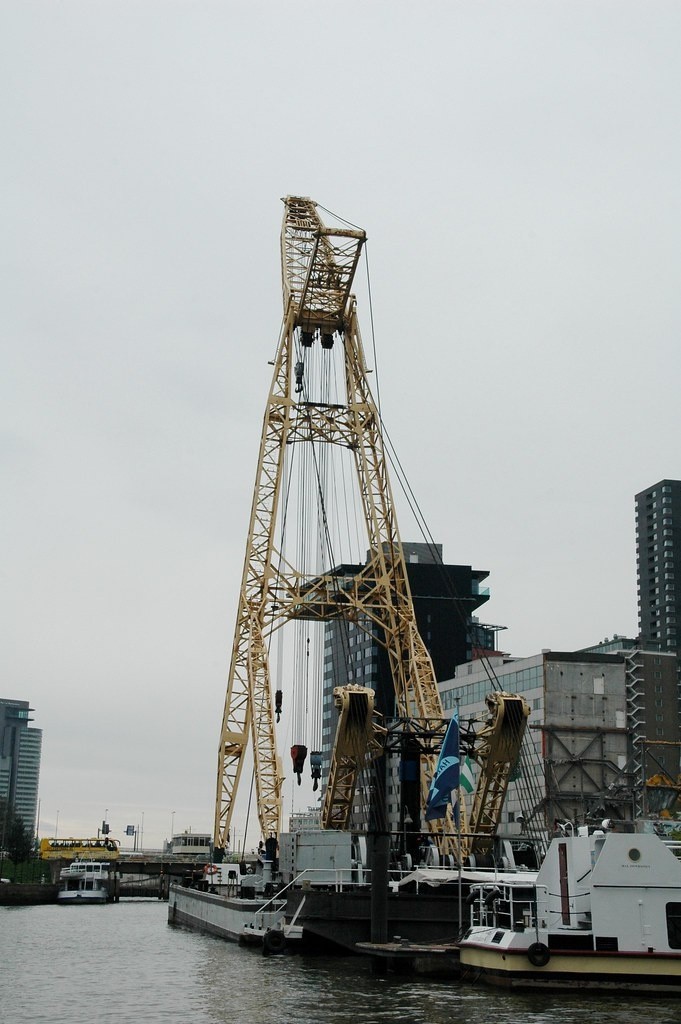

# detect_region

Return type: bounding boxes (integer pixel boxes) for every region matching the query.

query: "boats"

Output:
[57,860,123,904]
[460,823,681,994]
[168,878,546,969]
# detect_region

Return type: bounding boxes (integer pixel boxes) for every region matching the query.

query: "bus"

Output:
[40,837,121,860]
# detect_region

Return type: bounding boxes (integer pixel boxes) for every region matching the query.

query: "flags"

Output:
[424,705,459,831]
[460,750,476,793]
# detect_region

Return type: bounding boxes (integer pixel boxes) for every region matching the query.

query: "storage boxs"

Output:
[278,829,355,887]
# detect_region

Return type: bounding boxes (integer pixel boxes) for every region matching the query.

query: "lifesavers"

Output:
[205,864,218,874]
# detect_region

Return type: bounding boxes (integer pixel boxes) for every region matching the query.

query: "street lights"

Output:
[55,810,60,838]
[171,812,175,835]
[104,808,108,823]
[140,812,143,851]
[36,798,42,849]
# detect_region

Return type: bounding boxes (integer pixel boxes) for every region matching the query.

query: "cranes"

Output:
[200,195,532,871]
